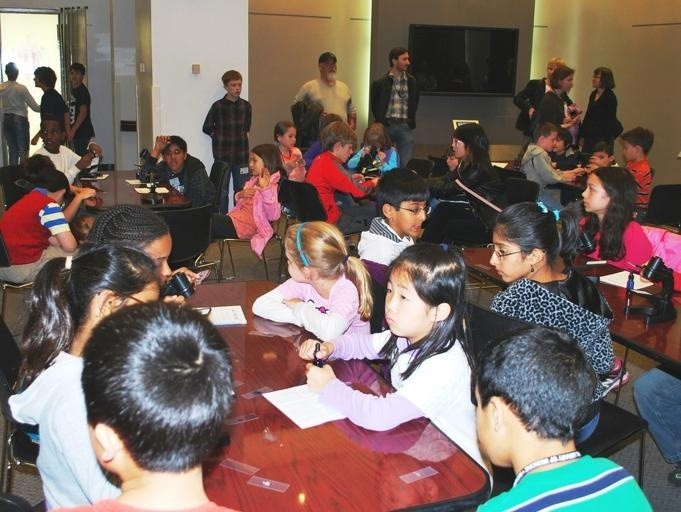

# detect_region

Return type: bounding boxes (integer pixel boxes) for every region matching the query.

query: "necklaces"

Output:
[511,451,583,487]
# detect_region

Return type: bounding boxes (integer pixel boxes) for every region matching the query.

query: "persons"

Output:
[31,66,73,146]
[66,63,97,155]
[570,166,655,399]
[68,214,97,241]
[519,119,584,213]
[4,155,96,225]
[589,144,619,167]
[250,221,374,343]
[305,121,381,234]
[0,170,93,283]
[138,135,217,208]
[291,52,358,131]
[470,324,654,512]
[418,145,469,214]
[578,66,617,153]
[32,114,102,184]
[274,120,307,219]
[302,113,343,170]
[586,126,655,224]
[489,201,615,446]
[631,363,680,488]
[348,121,400,177]
[80,202,203,308]
[0,63,41,165]
[528,64,582,144]
[197,144,282,263]
[46,300,237,511]
[371,48,418,168]
[550,128,577,169]
[298,241,494,511]
[8,244,162,511]
[411,122,514,244]
[202,70,252,213]
[513,54,565,158]
[349,168,431,332]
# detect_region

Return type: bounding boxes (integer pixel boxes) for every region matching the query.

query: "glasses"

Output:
[486,243,533,262]
[591,75,601,79]
[164,149,184,156]
[387,203,432,217]
[453,138,464,146]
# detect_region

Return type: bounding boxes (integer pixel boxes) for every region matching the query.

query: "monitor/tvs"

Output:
[406,21,520,98]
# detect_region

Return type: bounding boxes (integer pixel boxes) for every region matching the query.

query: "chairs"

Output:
[645,185,681,229]
[463,300,649,491]
[219,176,287,282]
[507,176,539,204]
[406,157,434,178]
[494,167,527,182]
[209,159,229,213]
[1,164,27,210]
[281,180,328,223]
[1,318,41,491]
[0,231,34,319]
[370,274,388,336]
[152,201,219,282]
[428,155,453,177]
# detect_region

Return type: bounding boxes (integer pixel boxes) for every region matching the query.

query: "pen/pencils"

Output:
[314,343,322,368]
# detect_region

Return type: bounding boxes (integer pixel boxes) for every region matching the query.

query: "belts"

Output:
[383,118,411,123]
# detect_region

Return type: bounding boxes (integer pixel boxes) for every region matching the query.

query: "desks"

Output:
[81,172,211,217]
[461,246,681,404]
[180,277,491,511]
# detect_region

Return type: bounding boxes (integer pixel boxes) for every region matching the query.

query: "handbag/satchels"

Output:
[0,283,33,338]
[614,118,624,140]
[195,237,284,285]
[272,210,298,242]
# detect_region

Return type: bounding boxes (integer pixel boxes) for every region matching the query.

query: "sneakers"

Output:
[668,462,681,488]
[601,356,629,399]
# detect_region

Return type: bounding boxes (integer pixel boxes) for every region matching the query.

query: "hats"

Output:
[319,52,337,63]
